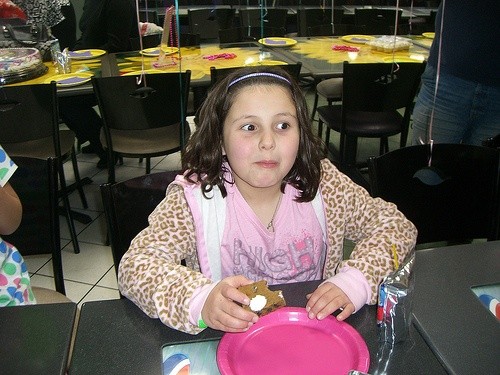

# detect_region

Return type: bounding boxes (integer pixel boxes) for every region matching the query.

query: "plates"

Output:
[342,34,377,44]
[44,72,93,87]
[140,47,179,56]
[422,32,435,38]
[258,37,298,47]
[217,307,371,375]
[68,49,106,59]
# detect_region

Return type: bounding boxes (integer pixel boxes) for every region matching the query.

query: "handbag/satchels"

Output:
[1,17,47,46]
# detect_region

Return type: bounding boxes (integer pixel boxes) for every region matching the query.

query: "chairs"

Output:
[0,0,500,299]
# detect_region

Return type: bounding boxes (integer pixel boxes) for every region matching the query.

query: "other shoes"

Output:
[97,156,117,169]
[81,144,96,153]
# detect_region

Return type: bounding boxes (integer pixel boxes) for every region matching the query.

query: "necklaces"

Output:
[266,196,282,229]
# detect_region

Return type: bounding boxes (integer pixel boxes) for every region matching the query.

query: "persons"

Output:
[118,66,416,334]
[51,0,134,169]
[413,0,500,145]
[0,145,36,306]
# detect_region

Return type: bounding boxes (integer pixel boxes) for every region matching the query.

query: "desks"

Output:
[0,240,500,375]
[268,35,430,174]
[0,53,113,224]
[109,41,312,114]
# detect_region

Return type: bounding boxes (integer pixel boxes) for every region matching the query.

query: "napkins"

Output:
[332,46,359,52]
[202,53,236,60]
